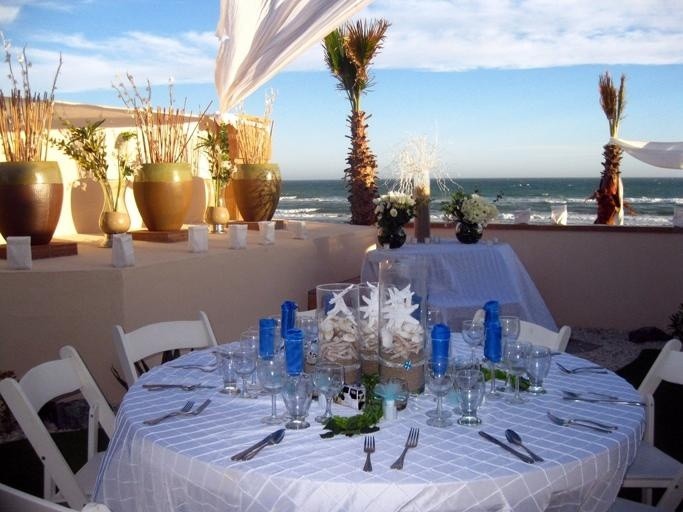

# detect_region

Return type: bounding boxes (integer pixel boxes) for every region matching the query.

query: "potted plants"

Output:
[196,114,231,236]
[47,119,136,247]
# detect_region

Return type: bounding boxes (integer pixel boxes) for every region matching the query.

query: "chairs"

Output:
[609,338,683,511]
[111,310,217,387]
[1,345,115,511]
[470,310,573,355]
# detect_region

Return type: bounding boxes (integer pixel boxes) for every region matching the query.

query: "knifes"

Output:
[170,362,217,368]
[142,384,217,389]
[564,399,646,405]
[231,429,284,461]
[480,430,534,465]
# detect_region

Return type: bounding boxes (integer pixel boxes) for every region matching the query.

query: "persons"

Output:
[471,189,479,199]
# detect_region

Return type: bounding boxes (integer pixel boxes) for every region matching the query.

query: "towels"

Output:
[484,300,502,364]
[410,294,421,323]
[284,329,304,376]
[325,293,335,316]
[280,300,296,338]
[430,323,451,375]
[257,318,277,358]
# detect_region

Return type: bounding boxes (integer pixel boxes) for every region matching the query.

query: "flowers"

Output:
[233,86,278,164]
[441,189,504,224]
[108,73,212,163]
[374,193,417,227]
[0,31,62,163]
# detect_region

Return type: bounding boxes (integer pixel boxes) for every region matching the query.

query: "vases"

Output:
[0,162,62,244]
[377,225,407,251]
[234,163,281,221]
[132,163,194,233]
[454,223,484,244]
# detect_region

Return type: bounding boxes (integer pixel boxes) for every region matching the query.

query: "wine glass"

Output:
[314,363,345,423]
[423,310,550,427]
[220,317,316,428]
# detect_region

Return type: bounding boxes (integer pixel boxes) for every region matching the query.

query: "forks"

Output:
[549,413,617,434]
[390,427,419,470]
[562,389,618,400]
[143,398,211,424]
[363,436,375,472]
[185,366,218,372]
[242,432,287,461]
[150,382,202,390]
[555,362,610,374]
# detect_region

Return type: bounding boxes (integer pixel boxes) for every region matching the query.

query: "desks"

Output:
[360,240,569,333]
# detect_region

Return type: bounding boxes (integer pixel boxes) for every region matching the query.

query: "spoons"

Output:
[506,429,543,462]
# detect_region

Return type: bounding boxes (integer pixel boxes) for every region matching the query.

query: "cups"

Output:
[378,260,426,396]
[317,284,362,384]
[390,378,409,409]
[360,282,395,376]
